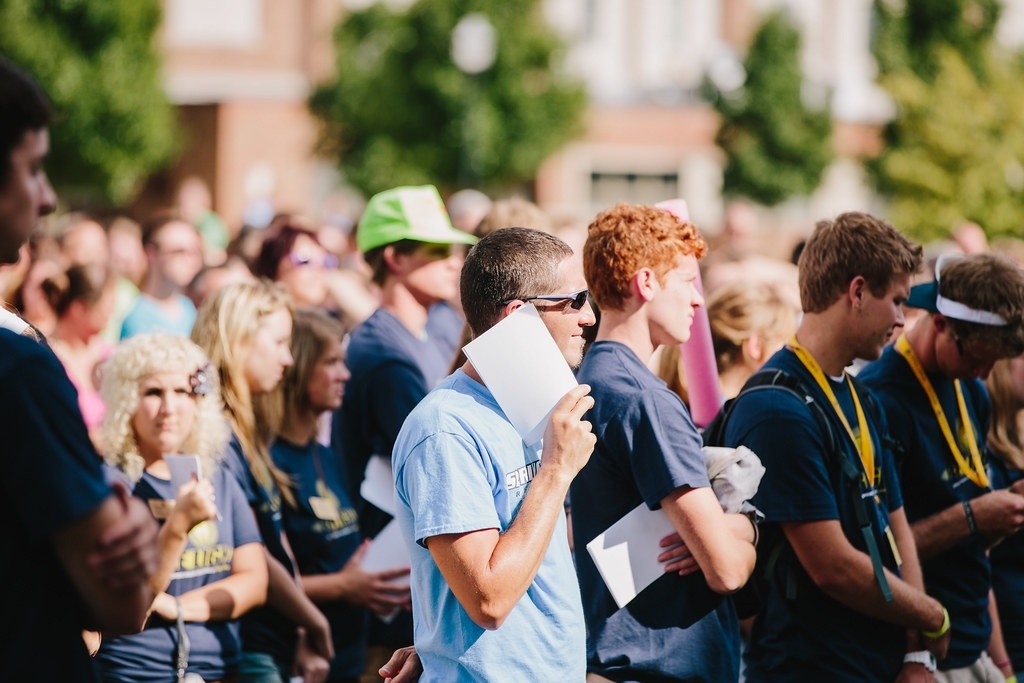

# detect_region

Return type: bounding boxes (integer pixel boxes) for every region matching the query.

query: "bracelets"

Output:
[750,519,760,547]
[963,500,977,534]
[922,607,951,638]
[998,659,1011,668]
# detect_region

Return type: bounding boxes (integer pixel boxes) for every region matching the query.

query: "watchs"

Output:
[904,649,937,673]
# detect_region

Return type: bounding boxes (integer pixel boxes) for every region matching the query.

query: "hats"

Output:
[358,185,481,256]
[904,253,1007,327]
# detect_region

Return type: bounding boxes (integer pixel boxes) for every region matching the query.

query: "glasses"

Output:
[496,287,588,310]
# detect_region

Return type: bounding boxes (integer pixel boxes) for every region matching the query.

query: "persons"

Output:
[390,226,601,682]
[575,205,765,683]
[0,160,1024,683]
[0,65,163,683]
